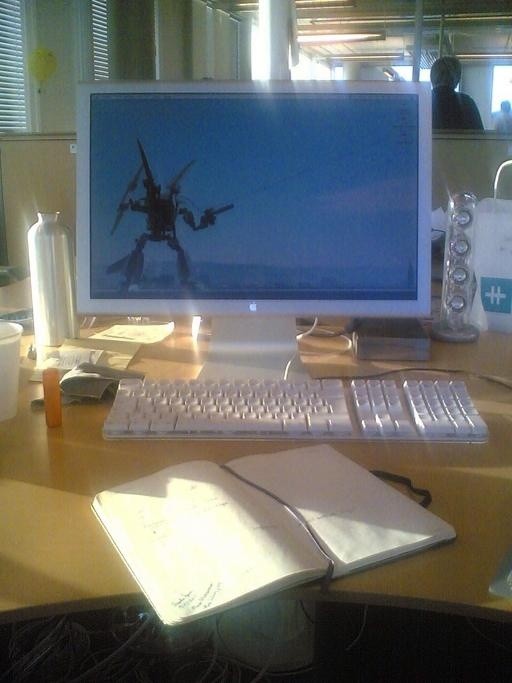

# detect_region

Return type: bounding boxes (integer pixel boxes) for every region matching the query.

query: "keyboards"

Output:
[100,377,492,444]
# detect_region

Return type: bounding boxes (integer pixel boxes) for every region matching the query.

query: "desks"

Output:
[0,313,512,625]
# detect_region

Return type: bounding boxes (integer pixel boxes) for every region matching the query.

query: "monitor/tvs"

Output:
[75,80,433,381]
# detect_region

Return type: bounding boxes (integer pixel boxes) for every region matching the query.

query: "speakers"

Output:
[429,190,479,345]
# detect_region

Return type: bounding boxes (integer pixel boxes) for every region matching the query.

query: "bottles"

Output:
[26,210,80,346]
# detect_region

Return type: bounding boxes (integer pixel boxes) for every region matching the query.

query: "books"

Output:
[90,445,458,627]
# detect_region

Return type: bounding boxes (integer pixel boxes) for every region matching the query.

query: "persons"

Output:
[495,101,512,135]
[430,56,484,130]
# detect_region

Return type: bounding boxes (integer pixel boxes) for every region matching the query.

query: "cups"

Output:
[0,319,24,422]
